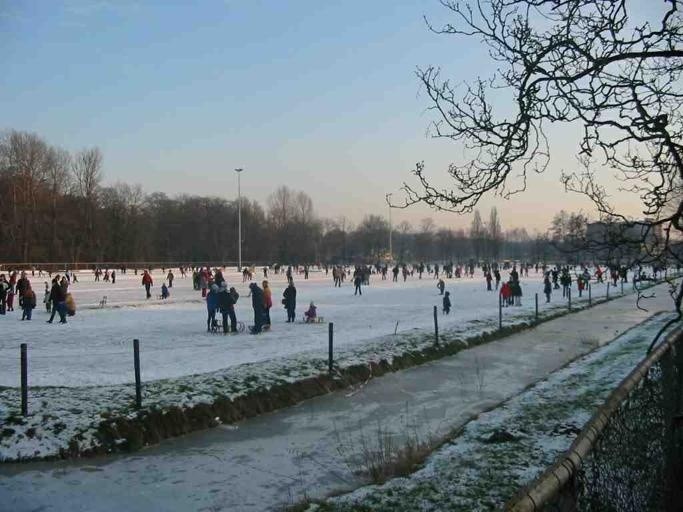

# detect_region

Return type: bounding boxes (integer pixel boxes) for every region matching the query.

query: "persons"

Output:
[93,264,225,299]
[205,280,273,334]
[544,277,551,303]
[443,291,452,314]
[503,262,669,297]
[1,264,78,324]
[242,262,501,295]
[499,277,522,307]
[283,280,296,322]
[304,302,316,323]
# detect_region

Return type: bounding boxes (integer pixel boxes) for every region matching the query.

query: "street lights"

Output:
[385,193,394,266]
[233,167,244,269]
